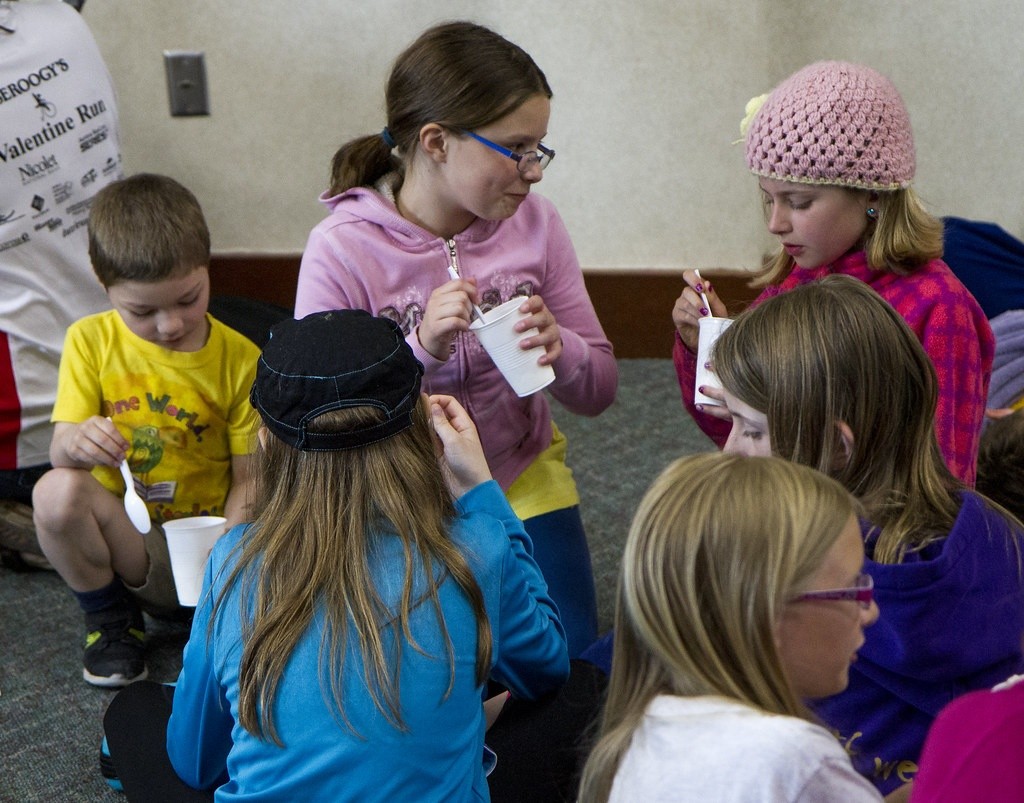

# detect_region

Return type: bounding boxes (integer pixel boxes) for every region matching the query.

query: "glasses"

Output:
[789,573,875,612]
[463,128,557,174]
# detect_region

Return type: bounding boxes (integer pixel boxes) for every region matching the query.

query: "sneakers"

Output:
[83,602,149,687]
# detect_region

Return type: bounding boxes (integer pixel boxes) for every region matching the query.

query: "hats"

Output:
[249,308,427,451]
[740,58,917,191]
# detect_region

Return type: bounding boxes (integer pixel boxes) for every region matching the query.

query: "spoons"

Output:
[106,417,149,535]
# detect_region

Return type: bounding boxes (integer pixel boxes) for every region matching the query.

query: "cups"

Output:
[470,295,556,398]
[163,516,229,608]
[694,317,736,408]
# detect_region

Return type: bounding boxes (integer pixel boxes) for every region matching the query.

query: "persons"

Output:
[670,57,998,497]
[291,20,619,668]
[480,271,1024,803]
[100,307,572,803]
[0,0,125,569]
[576,453,917,803]
[32,171,267,688]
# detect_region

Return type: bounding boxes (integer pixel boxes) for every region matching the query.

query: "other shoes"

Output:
[1,499,62,572]
